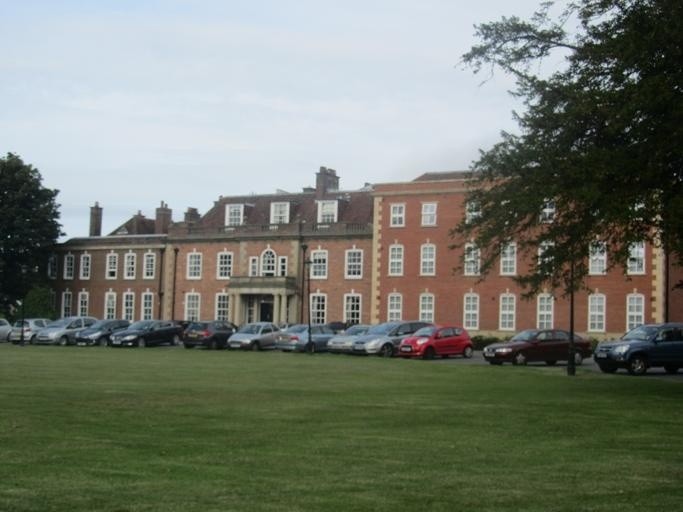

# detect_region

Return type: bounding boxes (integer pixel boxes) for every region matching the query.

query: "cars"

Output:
[483,328,592,365]
[593,322,683,374]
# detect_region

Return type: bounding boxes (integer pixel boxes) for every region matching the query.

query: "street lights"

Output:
[307,255,313,357]
[301,242,307,324]
